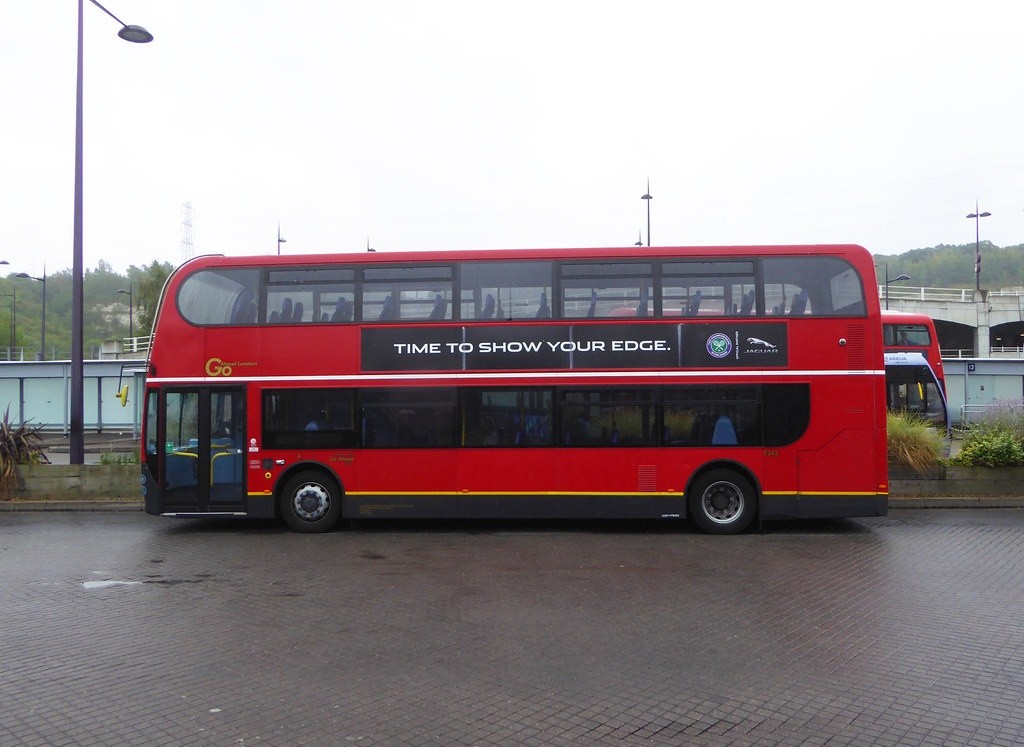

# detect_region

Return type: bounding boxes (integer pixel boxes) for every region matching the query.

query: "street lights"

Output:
[360,236,377,252]
[273,223,288,256]
[18,268,48,364]
[1,258,15,360]
[640,180,662,243]
[968,201,991,291]
[111,278,134,354]
[66,0,154,465]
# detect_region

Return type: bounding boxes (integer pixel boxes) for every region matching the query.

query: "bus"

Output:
[869,310,949,434]
[141,242,888,532]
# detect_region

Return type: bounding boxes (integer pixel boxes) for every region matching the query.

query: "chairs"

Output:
[899,338,908,345]
[509,423,674,446]
[230,289,809,323]
